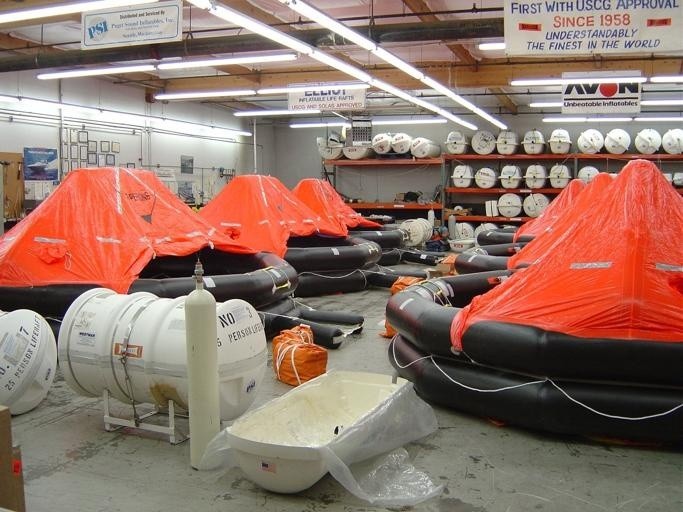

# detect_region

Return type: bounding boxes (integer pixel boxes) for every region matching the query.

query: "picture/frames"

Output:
[60,127,120,173]
[129,163,135,168]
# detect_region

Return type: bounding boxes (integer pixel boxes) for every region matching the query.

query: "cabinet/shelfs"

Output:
[446,154,576,222]
[578,154,682,197]
[324,154,444,226]
